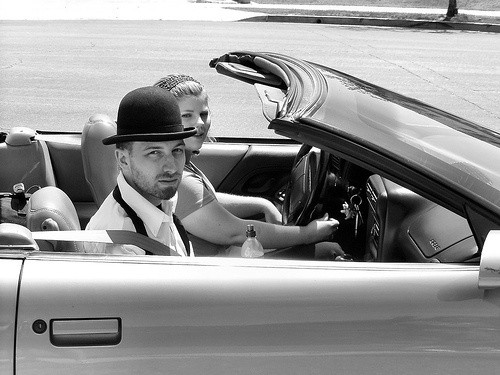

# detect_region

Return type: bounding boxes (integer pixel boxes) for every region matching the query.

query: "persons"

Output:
[153,74,340,256]
[83,86,346,261]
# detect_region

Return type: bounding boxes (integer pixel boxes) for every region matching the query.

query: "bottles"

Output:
[240,224,264,258]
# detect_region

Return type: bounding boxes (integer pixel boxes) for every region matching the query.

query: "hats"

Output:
[102,86,198,145]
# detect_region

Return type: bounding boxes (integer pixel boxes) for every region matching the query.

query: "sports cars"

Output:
[0,51,500,374]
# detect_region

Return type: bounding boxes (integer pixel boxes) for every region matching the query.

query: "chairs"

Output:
[27,114,117,252]
